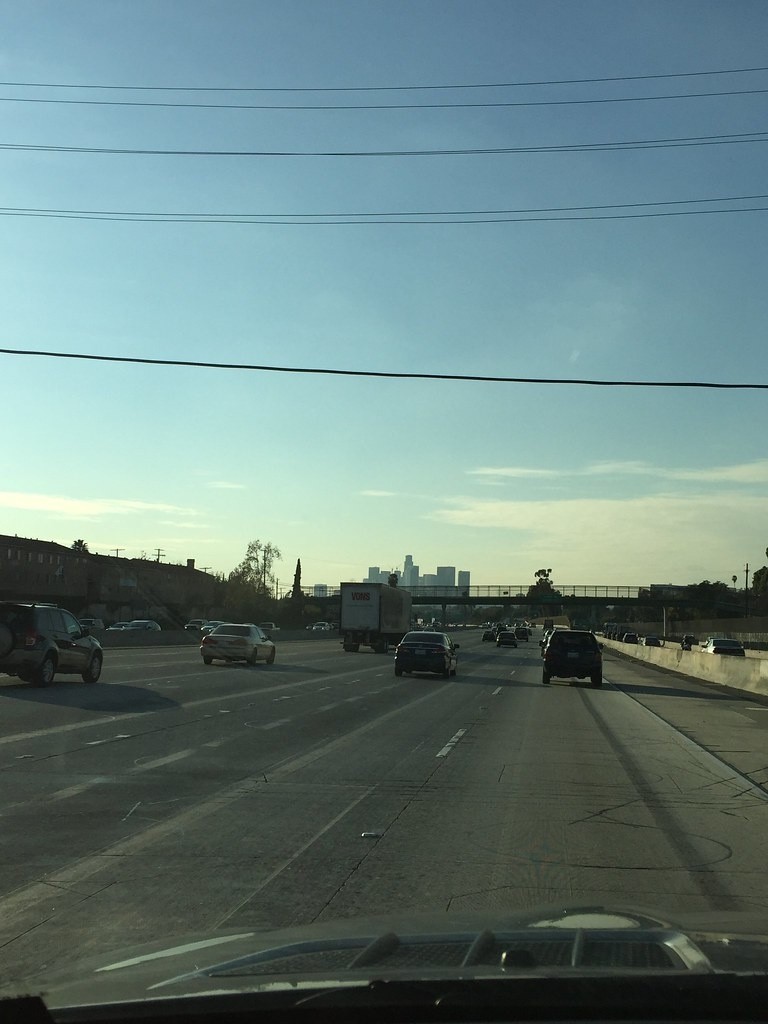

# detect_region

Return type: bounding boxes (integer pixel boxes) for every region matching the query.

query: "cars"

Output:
[621,632,638,644]
[544,630,553,637]
[393,631,460,678]
[515,628,528,641]
[122,620,161,632]
[201,621,224,630]
[184,619,208,630]
[481,618,536,633]
[643,636,660,647]
[106,622,130,632]
[539,629,605,684]
[543,628,553,636]
[0,900,768,1024]
[200,624,276,665]
[306,621,339,629]
[496,630,517,647]
[69,619,105,631]
[483,630,495,641]
[701,639,745,656]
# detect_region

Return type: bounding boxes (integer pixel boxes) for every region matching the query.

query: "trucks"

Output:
[339,583,418,653]
[603,622,632,642]
[544,619,553,628]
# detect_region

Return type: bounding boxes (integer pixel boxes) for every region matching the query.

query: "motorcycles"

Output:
[680,638,691,651]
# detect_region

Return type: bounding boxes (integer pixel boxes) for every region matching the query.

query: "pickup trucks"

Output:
[259,621,280,631]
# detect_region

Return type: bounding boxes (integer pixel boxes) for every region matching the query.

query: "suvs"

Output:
[0,601,103,684]
